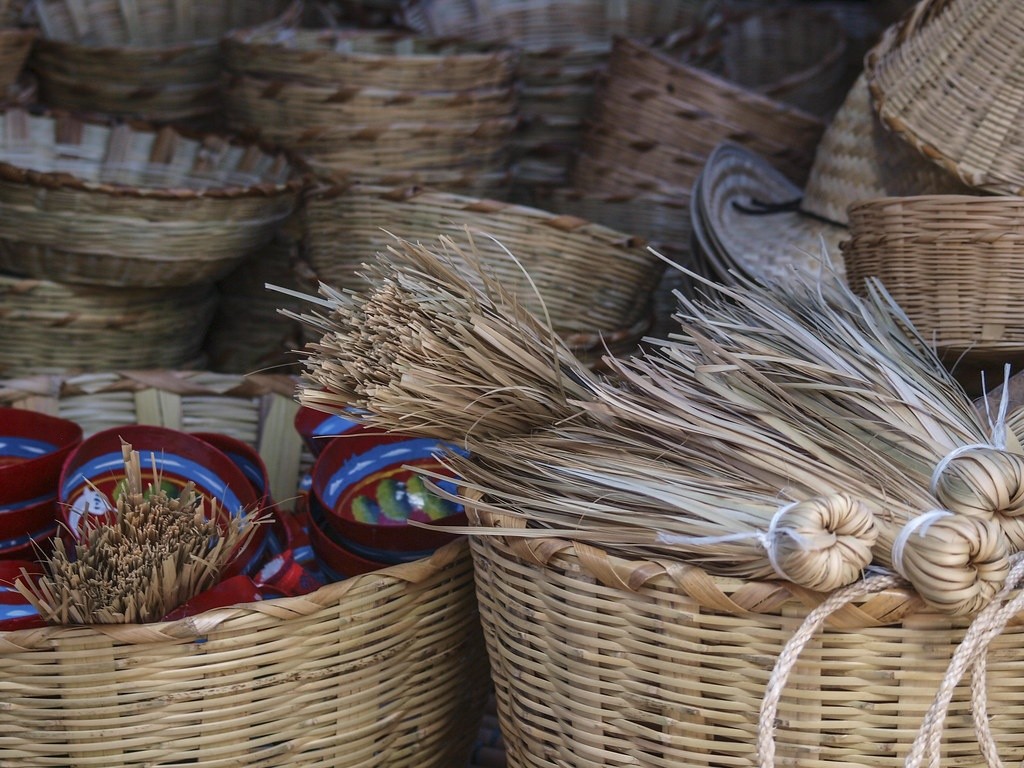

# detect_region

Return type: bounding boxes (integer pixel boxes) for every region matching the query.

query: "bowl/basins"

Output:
[0,388,470,642]
